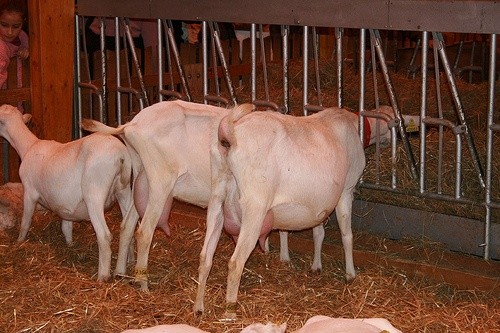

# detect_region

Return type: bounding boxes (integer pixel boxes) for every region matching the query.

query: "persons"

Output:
[0,0,30,114]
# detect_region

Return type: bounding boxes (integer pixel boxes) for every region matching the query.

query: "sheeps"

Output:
[0,104,138,284]
[81,100,401,323]
